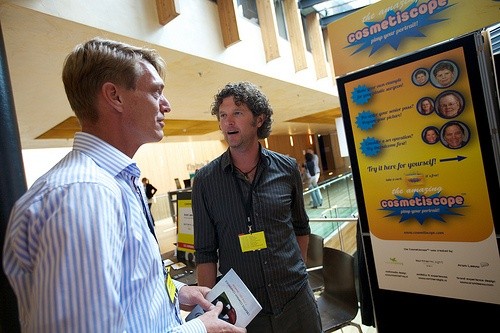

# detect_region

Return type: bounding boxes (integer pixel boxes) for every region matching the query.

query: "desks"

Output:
[166,188,192,224]
[162,249,222,312]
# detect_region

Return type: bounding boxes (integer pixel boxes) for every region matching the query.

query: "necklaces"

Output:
[233,162,259,178]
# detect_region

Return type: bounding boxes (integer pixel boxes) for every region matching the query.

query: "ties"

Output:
[131,176,167,275]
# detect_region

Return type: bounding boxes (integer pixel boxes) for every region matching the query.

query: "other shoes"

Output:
[311,206,317,209]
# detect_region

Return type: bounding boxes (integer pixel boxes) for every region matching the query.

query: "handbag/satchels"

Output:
[299,170,311,182]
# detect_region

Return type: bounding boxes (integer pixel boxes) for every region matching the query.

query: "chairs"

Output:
[305,234,324,291]
[317,247,363,333]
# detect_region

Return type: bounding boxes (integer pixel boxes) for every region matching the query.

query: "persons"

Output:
[420,99,433,115]
[432,62,455,85]
[2,39,248,333]
[424,129,439,144]
[415,70,427,85]
[305,147,322,209]
[191,81,323,333]
[443,123,467,149]
[438,92,461,118]
[142,178,157,226]
